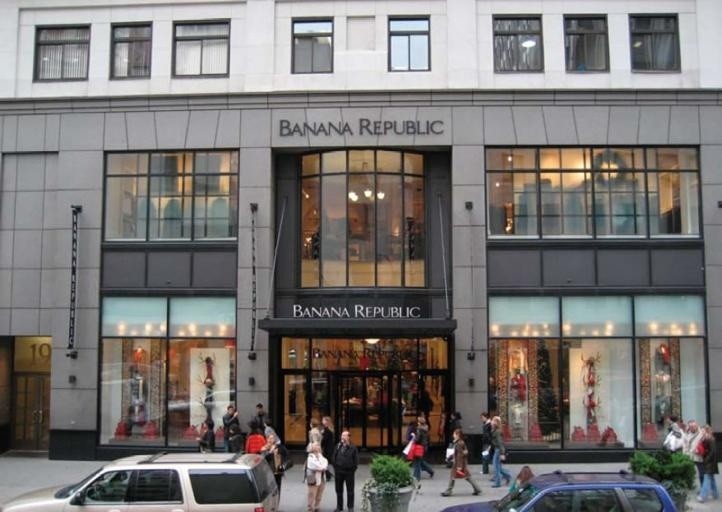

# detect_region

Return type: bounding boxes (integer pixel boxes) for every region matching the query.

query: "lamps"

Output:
[346,161,385,203]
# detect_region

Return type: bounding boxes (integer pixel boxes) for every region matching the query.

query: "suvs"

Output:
[531,407,561,437]
[439,470,678,512]
[0,451,280,512]
[168,387,237,426]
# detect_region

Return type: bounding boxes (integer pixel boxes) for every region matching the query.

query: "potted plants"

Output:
[626,442,698,512]
[360,453,418,512]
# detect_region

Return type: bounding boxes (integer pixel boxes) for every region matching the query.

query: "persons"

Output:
[350,375,389,427]
[481,412,511,487]
[223,403,293,491]
[655,371,673,412]
[441,413,482,496]
[130,376,147,419]
[305,417,359,511]
[512,369,526,425]
[133,345,147,375]
[662,412,722,504]
[392,380,435,495]
[196,420,215,452]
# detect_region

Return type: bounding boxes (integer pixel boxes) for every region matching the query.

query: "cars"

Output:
[288,376,329,392]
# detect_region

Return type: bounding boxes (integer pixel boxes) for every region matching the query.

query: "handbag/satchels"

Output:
[456,470,465,479]
[402,440,424,460]
